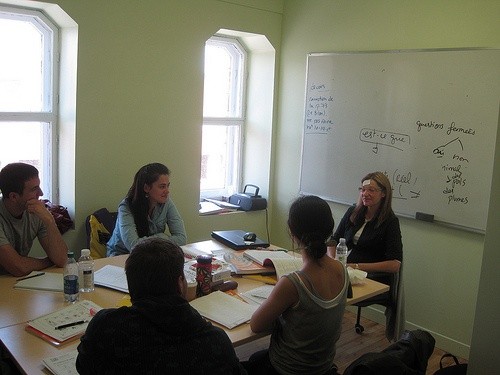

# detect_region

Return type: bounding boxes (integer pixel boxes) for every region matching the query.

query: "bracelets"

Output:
[355,262,359,269]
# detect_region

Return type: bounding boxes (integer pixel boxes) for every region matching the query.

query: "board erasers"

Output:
[415,212,435,224]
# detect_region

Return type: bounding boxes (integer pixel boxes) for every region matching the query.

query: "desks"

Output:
[0,240,389,375]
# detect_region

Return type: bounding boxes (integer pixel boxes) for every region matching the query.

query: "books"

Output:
[198,198,241,215]
[12,230,304,375]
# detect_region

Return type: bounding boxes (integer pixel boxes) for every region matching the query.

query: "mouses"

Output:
[243,232,257,241]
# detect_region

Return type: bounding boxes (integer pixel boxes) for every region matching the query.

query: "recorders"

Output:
[229,184,267,211]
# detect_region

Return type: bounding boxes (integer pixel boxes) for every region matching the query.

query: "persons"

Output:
[76,237,244,375]
[325,171,403,307]
[0,163,68,277]
[242,195,349,375]
[106,162,186,256]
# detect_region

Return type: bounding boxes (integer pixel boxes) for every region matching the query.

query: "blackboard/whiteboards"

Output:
[297,46,500,234]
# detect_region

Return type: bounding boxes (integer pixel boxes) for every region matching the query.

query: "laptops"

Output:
[211,230,270,251]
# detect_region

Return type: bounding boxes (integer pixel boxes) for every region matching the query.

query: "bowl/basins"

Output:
[347,268,367,285]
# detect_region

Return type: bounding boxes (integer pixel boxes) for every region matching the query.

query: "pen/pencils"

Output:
[16,272,45,281]
[55,319,87,329]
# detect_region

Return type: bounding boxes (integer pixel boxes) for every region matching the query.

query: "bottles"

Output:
[196,254,212,295]
[336,238,347,268]
[78,249,95,293]
[63,251,80,303]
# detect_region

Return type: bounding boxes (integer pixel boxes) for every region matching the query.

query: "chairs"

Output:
[86,212,118,249]
[342,295,435,375]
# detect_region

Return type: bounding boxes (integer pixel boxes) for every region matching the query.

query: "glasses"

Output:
[359,186,385,193]
